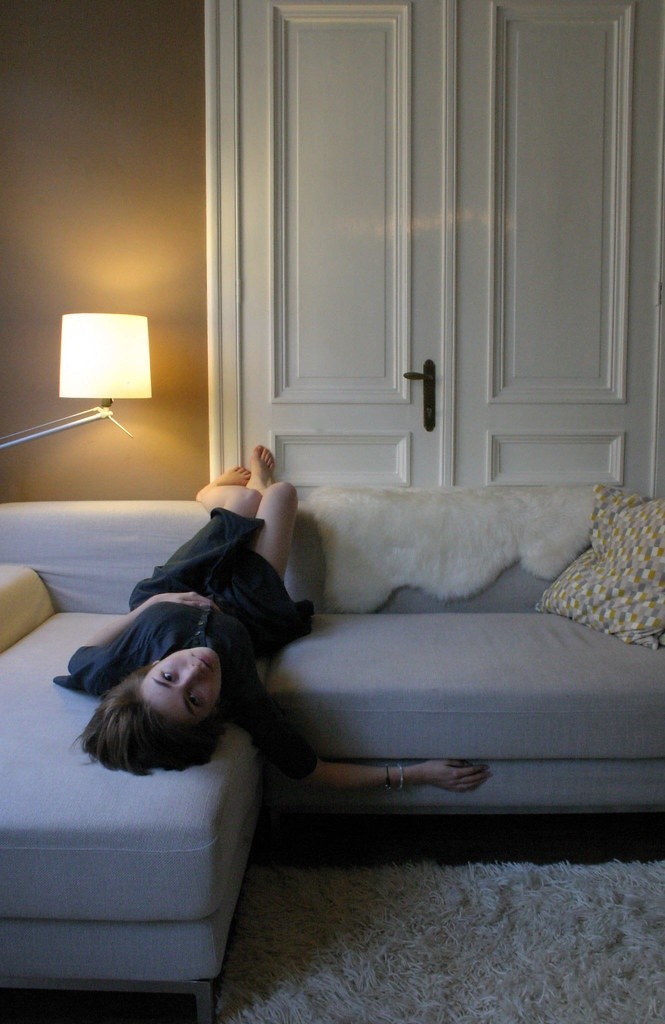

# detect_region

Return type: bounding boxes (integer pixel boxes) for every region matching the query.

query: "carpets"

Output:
[207,859,665,1024]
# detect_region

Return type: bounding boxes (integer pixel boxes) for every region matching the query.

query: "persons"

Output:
[50,445,490,796]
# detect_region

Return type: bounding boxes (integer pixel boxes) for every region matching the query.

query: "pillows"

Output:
[540,483,665,649]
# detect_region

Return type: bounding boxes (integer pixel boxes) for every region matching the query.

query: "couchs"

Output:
[0,484,665,1024]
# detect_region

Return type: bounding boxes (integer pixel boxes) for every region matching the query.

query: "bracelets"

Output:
[395,763,404,792]
[384,763,392,793]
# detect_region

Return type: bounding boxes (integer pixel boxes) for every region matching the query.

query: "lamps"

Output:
[0,313,152,449]
